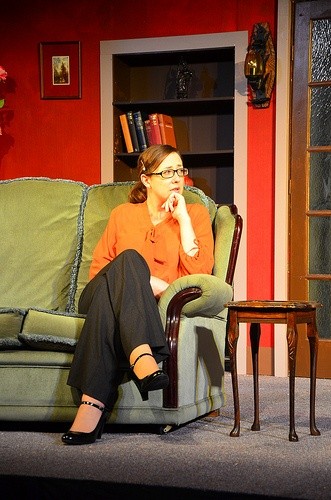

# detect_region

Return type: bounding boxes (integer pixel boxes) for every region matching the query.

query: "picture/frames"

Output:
[37,40,82,100]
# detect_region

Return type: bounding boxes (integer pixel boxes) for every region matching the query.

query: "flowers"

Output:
[0,64,8,136]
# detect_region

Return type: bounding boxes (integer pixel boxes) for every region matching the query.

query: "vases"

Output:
[173,53,193,99]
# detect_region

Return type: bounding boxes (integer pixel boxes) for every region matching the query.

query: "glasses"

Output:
[145,168,189,178]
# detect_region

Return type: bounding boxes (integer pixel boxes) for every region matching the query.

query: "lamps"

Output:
[243,22,276,109]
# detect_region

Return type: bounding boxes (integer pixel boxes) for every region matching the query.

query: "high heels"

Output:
[130,353,168,401]
[62,401,106,444]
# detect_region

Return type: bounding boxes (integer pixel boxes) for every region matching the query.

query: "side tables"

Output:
[223,299,324,442]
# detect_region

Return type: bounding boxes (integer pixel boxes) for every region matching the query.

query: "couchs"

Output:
[0,176,244,436]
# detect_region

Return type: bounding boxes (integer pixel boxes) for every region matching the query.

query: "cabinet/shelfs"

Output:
[111,45,235,205]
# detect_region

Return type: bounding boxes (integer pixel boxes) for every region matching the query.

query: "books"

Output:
[120,112,176,154]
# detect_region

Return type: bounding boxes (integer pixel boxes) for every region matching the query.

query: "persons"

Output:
[61,144,215,445]
